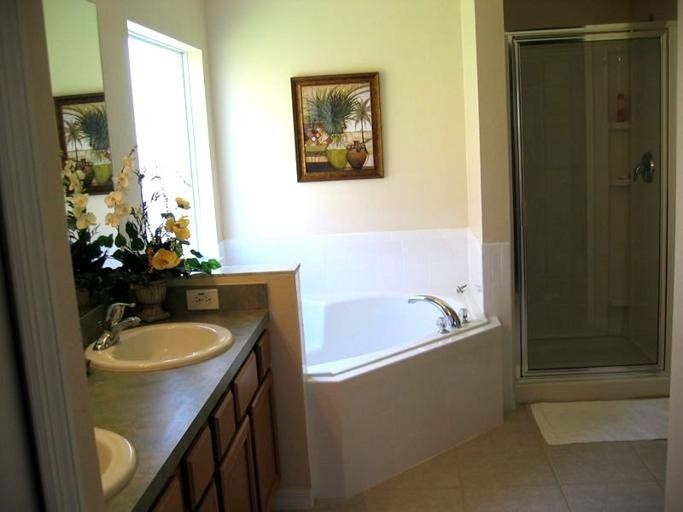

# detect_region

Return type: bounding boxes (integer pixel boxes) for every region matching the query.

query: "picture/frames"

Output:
[290,71,384,184]
[51,92,114,197]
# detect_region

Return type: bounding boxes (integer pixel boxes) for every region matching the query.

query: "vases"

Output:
[129,280,171,323]
[74,273,109,316]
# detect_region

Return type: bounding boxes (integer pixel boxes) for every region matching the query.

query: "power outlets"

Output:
[185,288,220,312]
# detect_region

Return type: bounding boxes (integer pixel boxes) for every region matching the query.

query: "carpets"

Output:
[529,396,669,445]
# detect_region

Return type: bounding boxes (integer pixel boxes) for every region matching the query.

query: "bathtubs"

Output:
[301,278,488,378]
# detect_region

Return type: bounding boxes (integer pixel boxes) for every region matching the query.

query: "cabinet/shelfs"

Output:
[144,330,284,512]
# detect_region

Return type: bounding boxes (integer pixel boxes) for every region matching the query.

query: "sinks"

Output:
[92,427,137,502]
[85,322,233,372]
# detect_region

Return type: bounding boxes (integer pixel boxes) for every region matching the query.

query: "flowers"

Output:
[58,150,114,276]
[104,142,223,289]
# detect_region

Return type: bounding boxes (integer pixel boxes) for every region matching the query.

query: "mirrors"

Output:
[40,0,125,318]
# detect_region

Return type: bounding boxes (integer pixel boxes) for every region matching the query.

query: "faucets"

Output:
[93,302,141,350]
[407,295,462,327]
